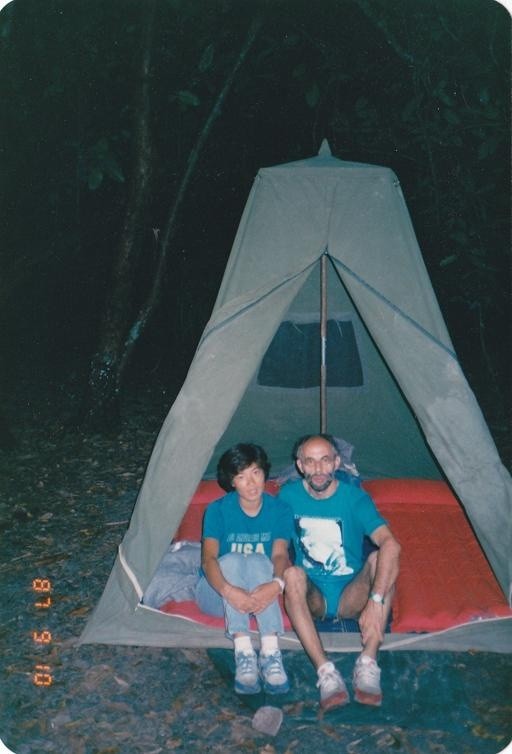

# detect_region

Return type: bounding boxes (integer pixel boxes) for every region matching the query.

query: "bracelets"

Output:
[273,577,286,596]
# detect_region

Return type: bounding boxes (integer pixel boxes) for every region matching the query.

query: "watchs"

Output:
[369,593,385,605]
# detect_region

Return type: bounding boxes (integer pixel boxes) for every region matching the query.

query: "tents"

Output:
[77,139,512,655]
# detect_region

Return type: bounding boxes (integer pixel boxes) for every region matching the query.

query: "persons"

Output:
[276,434,402,712]
[194,446,295,697]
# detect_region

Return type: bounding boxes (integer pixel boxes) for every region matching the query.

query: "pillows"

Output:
[359,479,456,508]
[187,475,281,507]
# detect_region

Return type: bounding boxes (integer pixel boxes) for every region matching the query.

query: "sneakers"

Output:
[315,660,350,713]
[233,648,261,696]
[258,648,290,696]
[351,656,382,708]
